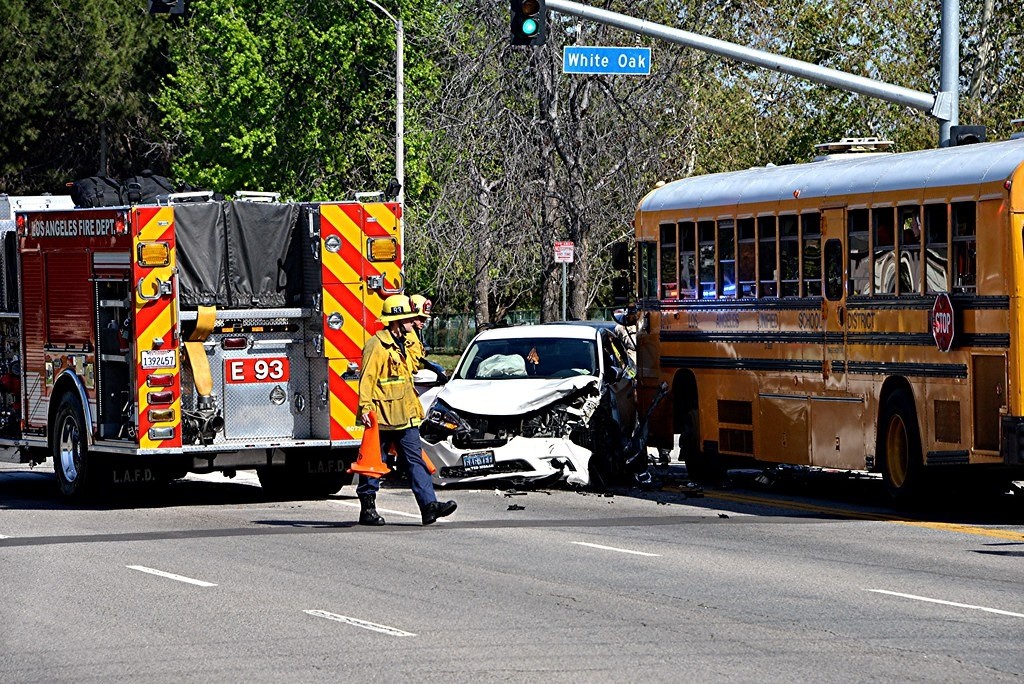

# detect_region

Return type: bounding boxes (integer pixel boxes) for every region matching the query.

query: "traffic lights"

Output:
[509,0,545,45]
[950,125,986,147]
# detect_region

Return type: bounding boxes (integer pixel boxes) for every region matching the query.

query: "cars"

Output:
[415,321,648,491]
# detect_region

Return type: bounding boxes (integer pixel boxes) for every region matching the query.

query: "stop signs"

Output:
[932,293,955,353]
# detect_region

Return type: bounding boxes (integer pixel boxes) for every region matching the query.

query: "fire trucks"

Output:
[0,177,405,504]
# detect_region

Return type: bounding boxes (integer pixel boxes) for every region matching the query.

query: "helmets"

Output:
[376,294,420,322]
[411,294,432,318]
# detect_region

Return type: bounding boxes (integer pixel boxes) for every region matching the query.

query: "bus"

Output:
[613,136,1024,522]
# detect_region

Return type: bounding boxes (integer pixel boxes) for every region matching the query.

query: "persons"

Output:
[356,295,457,526]
[613,306,640,368]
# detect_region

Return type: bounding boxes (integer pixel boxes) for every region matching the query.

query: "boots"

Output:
[420,500,458,525]
[357,492,386,526]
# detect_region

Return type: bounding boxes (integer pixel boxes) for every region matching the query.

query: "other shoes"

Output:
[659,453,671,462]
